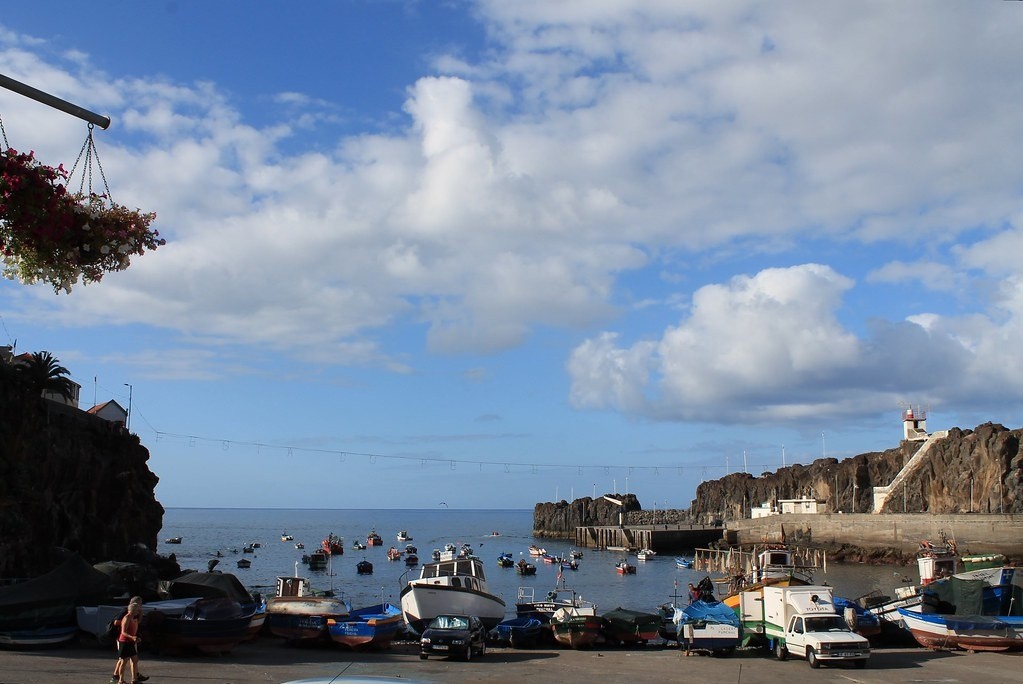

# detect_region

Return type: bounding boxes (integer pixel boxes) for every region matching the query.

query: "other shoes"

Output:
[118,681,129,684]
[130,681,143,684]
[110,675,119,682]
[134,674,149,682]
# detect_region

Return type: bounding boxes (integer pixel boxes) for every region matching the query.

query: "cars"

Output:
[710,519,722,526]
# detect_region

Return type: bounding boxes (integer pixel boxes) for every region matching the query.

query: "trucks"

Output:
[738,586,871,668]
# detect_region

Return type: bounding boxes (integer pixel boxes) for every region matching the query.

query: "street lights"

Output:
[124,384,132,431]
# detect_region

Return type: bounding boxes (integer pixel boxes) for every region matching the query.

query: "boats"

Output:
[397,551,506,635]
[497,549,660,647]
[280,528,293,541]
[165,536,183,544]
[513,559,537,575]
[496,551,514,568]
[295,542,304,549]
[615,558,636,575]
[658,522,1023,651]
[634,549,656,561]
[528,544,584,571]
[356,556,374,575]
[76,549,406,651]
[431,543,474,560]
[302,531,345,571]
[242,542,260,552]
[237,559,251,568]
[353,530,419,565]
[674,553,693,569]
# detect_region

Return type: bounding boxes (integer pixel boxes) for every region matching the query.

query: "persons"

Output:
[234,549,238,552]
[243,546,246,550]
[806,525,811,535]
[109,595,150,684]
[545,587,557,601]
[734,570,747,589]
[250,544,253,548]
[161,552,180,592]
[688,583,698,604]
[217,550,221,557]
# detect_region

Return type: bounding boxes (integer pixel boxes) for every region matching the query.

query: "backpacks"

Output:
[106,611,125,635]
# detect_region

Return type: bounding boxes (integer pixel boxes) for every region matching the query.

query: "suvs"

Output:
[419,613,486,662]
